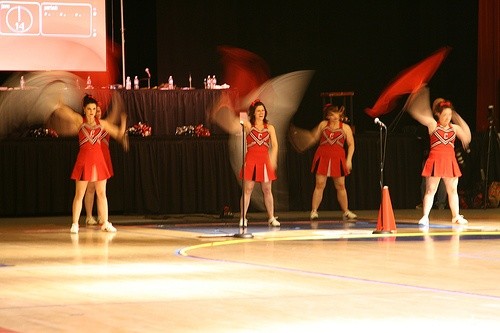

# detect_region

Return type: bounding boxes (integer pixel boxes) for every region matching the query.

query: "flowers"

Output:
[440,101,452,106]
[252,99,260,107]
[322,104,332,112]
[30,128,58,139]
[128,122,153,137]
[175,124,210,137]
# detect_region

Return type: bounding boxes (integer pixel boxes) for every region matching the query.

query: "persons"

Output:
[232,99,281,227]
[302,104,358,219]
[410,96,472,225]
[54,90,126,233]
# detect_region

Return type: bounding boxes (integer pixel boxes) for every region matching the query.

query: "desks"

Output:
[0,130,500,216]
[0,89,235,132]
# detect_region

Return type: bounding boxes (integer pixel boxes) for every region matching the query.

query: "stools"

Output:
[321,92,354,127]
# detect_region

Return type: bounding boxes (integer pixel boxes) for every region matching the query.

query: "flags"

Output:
[367,51,447,115]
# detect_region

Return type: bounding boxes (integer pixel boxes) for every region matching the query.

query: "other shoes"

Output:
[452,214,468,225]
[100,222,117,232]
[70,223,80,234]
[418,216,429,225]
[85,218,97,225]
[416,204,423,209]
[310,211,319,220]
[439,205,444,211]
[342,210,358,219]
[239,218,248,227]
[268,216,281,227]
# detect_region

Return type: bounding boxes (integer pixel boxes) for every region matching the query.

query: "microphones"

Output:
[488,105,496,119]
[373,118,388,130]
[240,121,245,126]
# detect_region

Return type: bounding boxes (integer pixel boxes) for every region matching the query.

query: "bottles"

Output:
[20,76,25,90]
[204,75,217,89]
[168,76,174,90]
[126,76,131,90]
[87,76,92,90]
[134,76,139,90]
